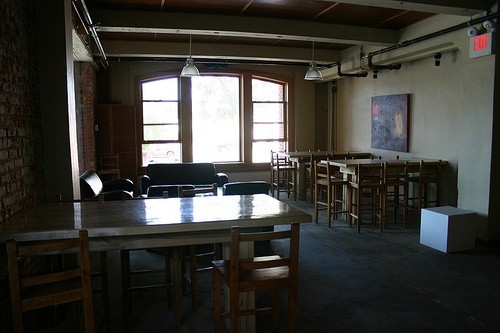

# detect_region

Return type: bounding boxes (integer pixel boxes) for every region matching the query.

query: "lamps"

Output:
[179,35,201,77]
[332,81,337,92]
[373,69,378,79]
[433,53,441,66]
[468,20,496,59]
[304,41,323,81]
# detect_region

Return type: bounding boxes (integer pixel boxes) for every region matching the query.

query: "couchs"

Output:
[80,170,135,201]
[223,180,274,253]
[139,164,228,195]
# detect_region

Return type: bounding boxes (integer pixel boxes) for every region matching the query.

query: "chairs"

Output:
[6,150,441,333]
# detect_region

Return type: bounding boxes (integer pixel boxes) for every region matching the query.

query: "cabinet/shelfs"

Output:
[94,104,138,175]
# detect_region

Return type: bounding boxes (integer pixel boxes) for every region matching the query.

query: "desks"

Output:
[420,206,477,254]
[321,158,448,225]
[276,151,372,200]
[0,191,312,330]
